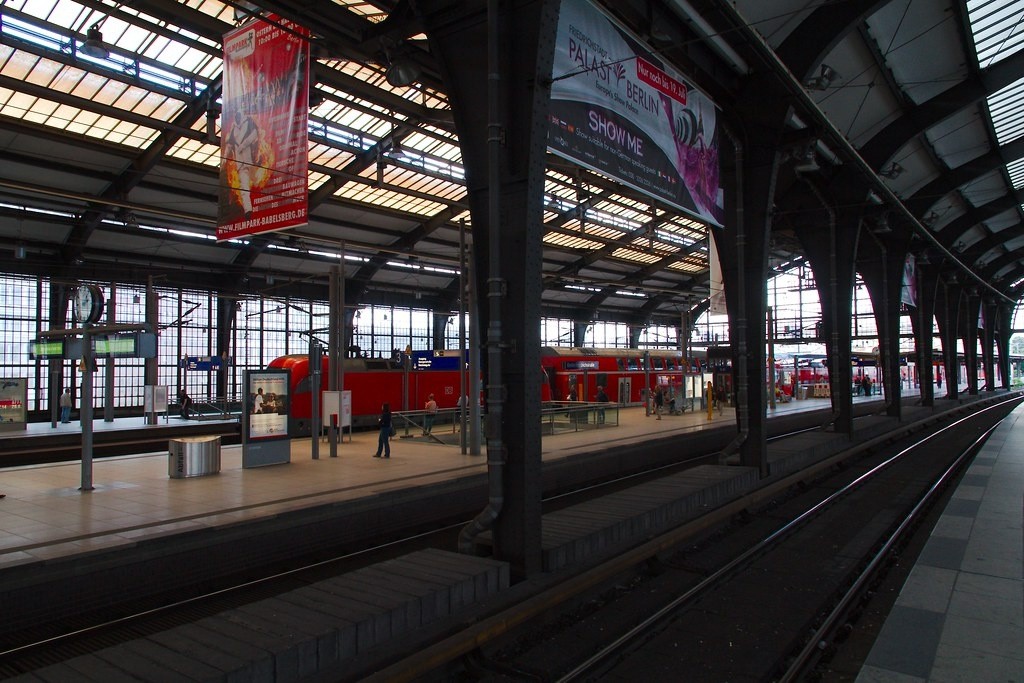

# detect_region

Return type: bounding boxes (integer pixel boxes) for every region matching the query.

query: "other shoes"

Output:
[381,455,386,459]
[373,455,378,457]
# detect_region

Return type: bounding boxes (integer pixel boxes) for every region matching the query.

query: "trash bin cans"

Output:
[168,435,221,478]
[802,385,814,400]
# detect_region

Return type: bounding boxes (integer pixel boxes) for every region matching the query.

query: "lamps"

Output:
[381,47,420,88]
[297,242,308,254]
[953,241,966,251]
[989,275,1003,284]
[773,265,784,272]
[14,246,25,260]
[916,256,931,265]
[973,260,985,269]
[415,275,422,300]
[126,214,139,228]
[947,275,958,285]
[804,64,843,91]
[78,24,108,59]
[968,289,980,297]
[872,218,892,234]
[794,152,820,172]
[922,211,940,224]
[879,162,908,179]
[265,253,274,286]
[986,298,997,306]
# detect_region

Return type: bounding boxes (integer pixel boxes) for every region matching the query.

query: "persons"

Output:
[715,386,727,416]
[819,374,824,383]
[937,373,942,389]
[255,388,263,414]
[855,375,872,396]
[423,394,436,435]
[222,110,261,218]
[649,385,676,420]
[372,404,392,459]
[594,386,605,424]
[60,388,72,423]
[458,395,468,431]
[564,385,576,417]
[180,390,190,420]
[267,394,276,411]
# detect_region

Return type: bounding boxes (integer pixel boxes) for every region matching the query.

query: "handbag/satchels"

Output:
[389,425,396,436]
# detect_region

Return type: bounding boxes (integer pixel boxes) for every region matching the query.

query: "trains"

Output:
[264,341,871,439]
